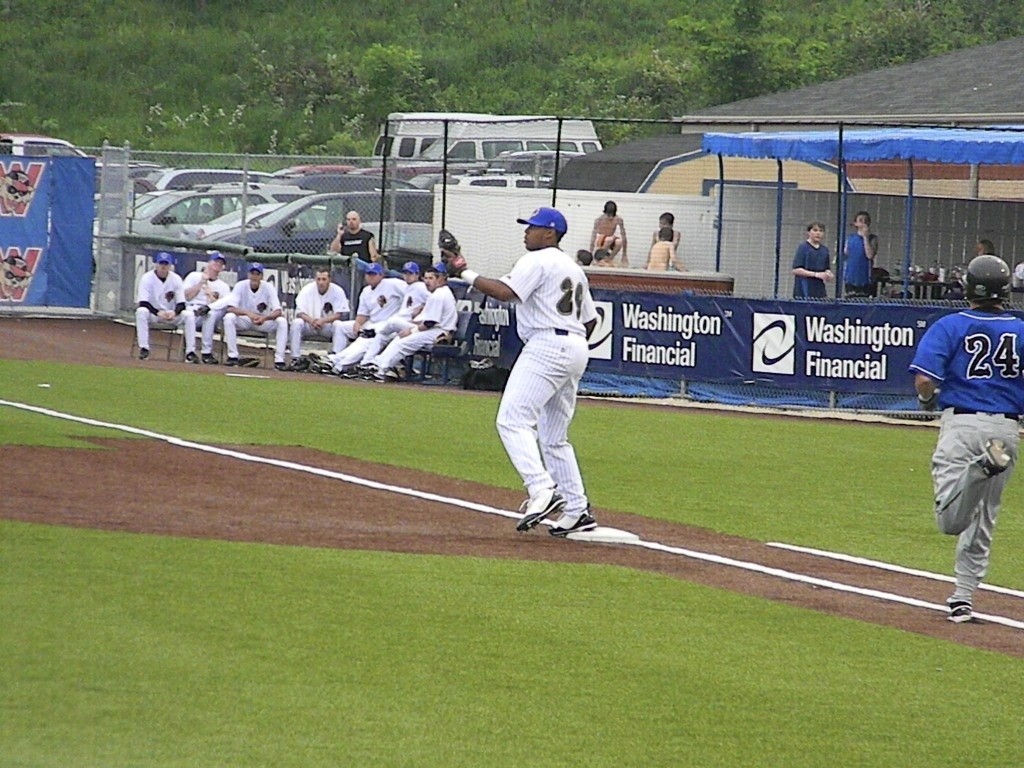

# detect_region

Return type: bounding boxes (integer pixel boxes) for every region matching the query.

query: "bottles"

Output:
[892,258,902,278]
[908,264,946,283]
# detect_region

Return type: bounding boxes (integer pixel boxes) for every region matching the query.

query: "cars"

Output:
[0,131,589,266]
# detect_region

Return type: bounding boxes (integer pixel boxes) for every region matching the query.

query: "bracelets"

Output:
[461,269,478,285]
[917,395,933,404]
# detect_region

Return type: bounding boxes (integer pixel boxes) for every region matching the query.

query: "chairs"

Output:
[130,324,332,370]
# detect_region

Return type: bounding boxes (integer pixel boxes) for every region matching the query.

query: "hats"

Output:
[432,261,448,274]
[247,262,264,273]
[364,262,385,276]
[209,252,226,264]
[516,206,567,234]
[402,261,420,274]
[156,252,172,265]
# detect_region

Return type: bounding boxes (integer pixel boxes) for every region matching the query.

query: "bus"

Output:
[370,112,603,170]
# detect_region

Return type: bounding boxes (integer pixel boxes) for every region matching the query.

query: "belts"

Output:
[951,405,1020,422]
[555,328,568,335]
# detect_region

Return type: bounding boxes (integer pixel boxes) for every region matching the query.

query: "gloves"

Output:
[918,389,939,412]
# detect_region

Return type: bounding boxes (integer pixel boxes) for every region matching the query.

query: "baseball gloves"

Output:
[237,357,260,367]
[438,229,468,279]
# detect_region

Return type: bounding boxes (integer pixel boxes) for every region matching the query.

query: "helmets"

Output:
[962,255,1013,303]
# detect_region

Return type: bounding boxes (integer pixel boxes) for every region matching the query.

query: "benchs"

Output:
[404,310,478,385]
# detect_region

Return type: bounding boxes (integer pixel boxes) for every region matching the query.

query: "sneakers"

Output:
[201,354,218,365]
[137,348,151,361]
[946,601,974,624]
[274,362,289,372]
[309,352,334,365]
[309,363,333,375]
[360,371,387,382]
[516,487,568,532]
[227,356,240,367]
[985,436,1016,468]
[548,502,598,537]
[184,351,199,363]
[355,362,380,372]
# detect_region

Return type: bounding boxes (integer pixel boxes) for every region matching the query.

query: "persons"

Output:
[342,261,429,380]
[943,240,994,300]
[909,256,1024,624]
[135,252,200,364]
[354,263,457,382]
[576,201,629,267]
[792,222,834,302]
[291,269,350,370]
[837,211,879,295]
[183,253,230,364]
[222,262,288,371]
[644,213,687,271]
[308,263,411,376]
[329,211,380,264]
[441,208,596,536]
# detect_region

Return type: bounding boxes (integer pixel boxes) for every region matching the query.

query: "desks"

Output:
[888,275,962,299]
